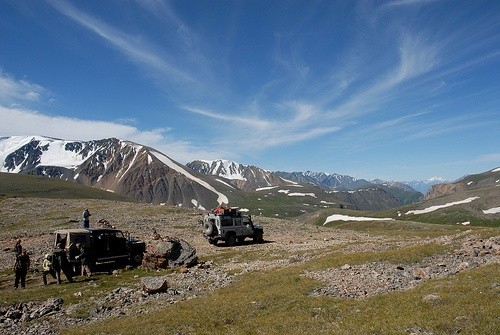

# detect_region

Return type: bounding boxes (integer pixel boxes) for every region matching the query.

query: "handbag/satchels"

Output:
[14,257,22,273]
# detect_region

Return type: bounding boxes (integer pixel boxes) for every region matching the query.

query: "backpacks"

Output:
[82,211,88,218]
[14,243,18,252]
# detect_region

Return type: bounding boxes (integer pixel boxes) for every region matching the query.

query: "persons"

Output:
[12,249,30,290]
[42,209,92,286]
[14,239,22,270]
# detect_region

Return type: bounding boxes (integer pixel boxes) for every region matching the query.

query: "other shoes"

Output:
[21,287,25,289]
[14,287,17,289]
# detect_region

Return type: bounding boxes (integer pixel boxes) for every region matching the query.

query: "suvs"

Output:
[203,212,264,245]
[55,228,147,268]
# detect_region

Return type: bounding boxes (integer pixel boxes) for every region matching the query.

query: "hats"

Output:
[16,239,21,242]
[45,254,49,258]
